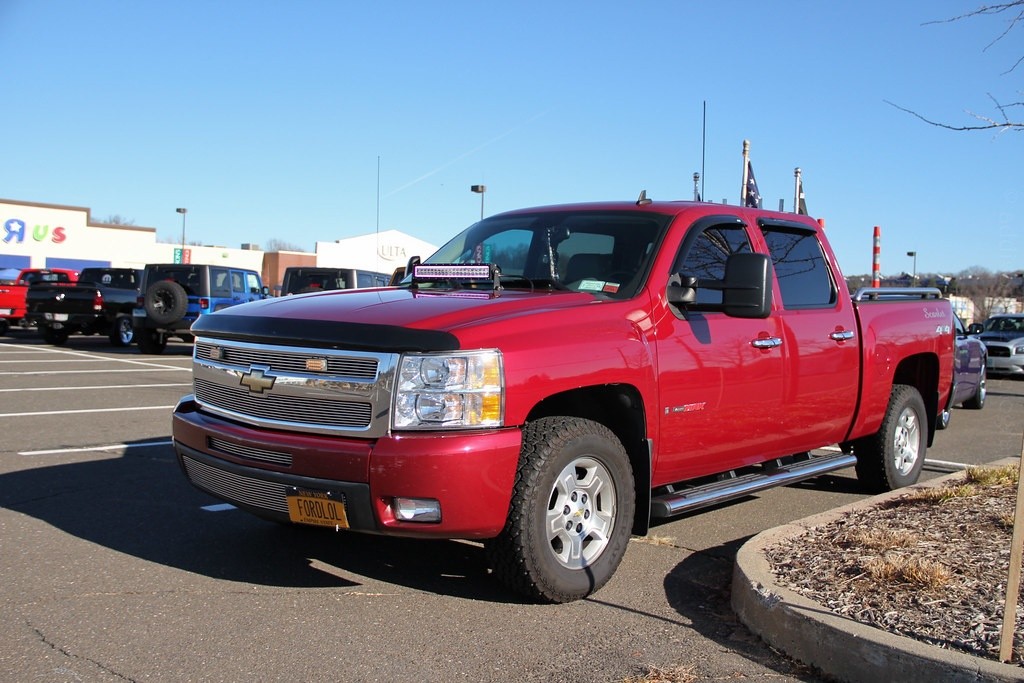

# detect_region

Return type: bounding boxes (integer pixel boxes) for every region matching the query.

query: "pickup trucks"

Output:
[0,268,75,344]
[22,263,144,346]
[172,198,956,606]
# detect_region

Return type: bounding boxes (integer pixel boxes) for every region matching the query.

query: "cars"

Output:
[852,288,989,430]
[975,313,1024,380]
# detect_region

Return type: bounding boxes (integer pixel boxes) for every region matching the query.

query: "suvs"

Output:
[132,261,270,353]
[281,262,396,300]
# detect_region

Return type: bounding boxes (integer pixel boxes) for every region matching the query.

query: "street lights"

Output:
[471,183,487,221]
[906,252,917,288]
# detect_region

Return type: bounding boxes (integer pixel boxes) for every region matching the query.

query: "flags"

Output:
[747,162,763,208]
[799,183,808,216]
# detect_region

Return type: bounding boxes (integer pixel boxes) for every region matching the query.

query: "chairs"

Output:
[563,254,614,286]
[222,275,236,291]
[325,281,337,291]
